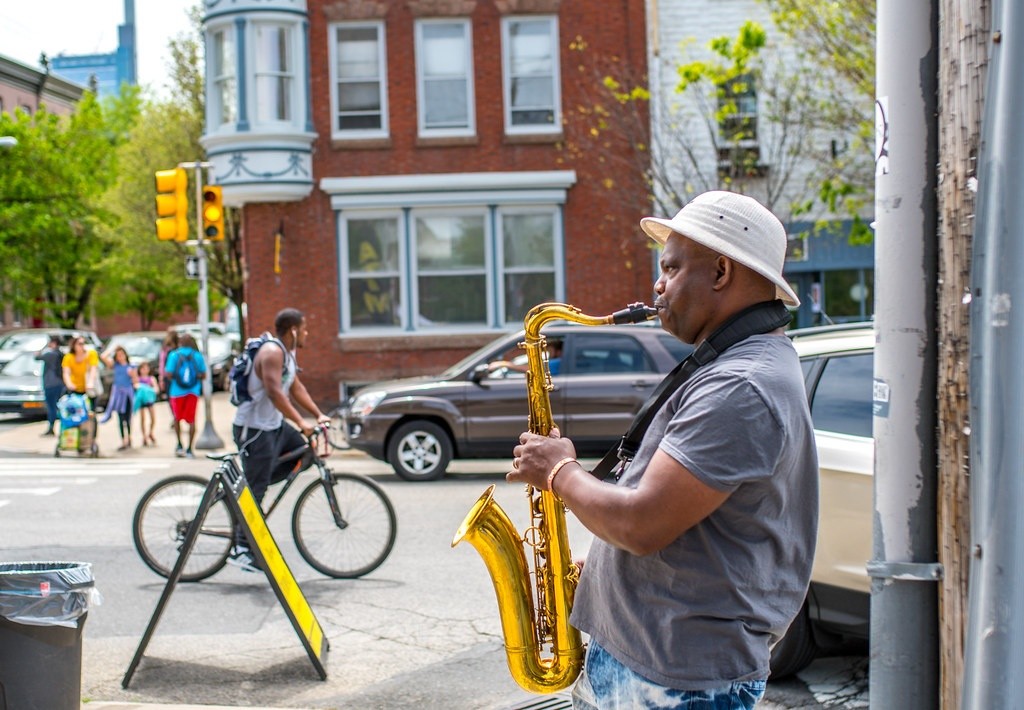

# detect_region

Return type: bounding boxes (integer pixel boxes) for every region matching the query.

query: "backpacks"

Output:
[173,348,197,388]
[228,331,298,406]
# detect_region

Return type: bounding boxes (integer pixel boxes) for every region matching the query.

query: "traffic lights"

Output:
[201,185,225,242]
[154,168,189,243]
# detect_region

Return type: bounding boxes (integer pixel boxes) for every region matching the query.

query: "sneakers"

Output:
[225,546,263,574]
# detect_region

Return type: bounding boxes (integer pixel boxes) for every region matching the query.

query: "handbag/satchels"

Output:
[85,370,104,398]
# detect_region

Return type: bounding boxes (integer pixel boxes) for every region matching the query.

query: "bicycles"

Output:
[133,421,398,582]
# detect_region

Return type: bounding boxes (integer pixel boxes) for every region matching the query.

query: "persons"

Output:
[35,336,66,436]
[100,345,139,451]
[61,337,98,456]
[506,191,820,710]
[158,331,180,430]
[163,334,207,458]
[226,307,331,574]
[135,364,161,447]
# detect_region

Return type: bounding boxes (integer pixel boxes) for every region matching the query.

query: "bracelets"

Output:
[547,457,581,507]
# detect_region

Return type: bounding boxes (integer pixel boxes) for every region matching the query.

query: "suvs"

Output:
[343,319,696,483]
[766,321,875,682]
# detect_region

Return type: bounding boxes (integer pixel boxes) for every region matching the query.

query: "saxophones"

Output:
[450,299,660,697]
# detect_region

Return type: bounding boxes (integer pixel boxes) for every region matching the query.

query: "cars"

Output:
[168,303,247,392]
[0,328,106,421]
[94,331,176,410]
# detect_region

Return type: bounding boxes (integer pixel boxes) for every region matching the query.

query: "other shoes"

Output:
[118,447,125,451]
[149,434,155,442]
[186,449,193,457]
[142,442,147,447]
[176,444,182,452]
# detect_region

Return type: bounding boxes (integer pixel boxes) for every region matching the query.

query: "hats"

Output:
[640,190,801,307]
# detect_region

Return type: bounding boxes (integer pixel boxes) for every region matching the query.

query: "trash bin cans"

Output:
[0,562,95,710]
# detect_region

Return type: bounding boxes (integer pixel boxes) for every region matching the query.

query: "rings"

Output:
[515,458,519,468]
